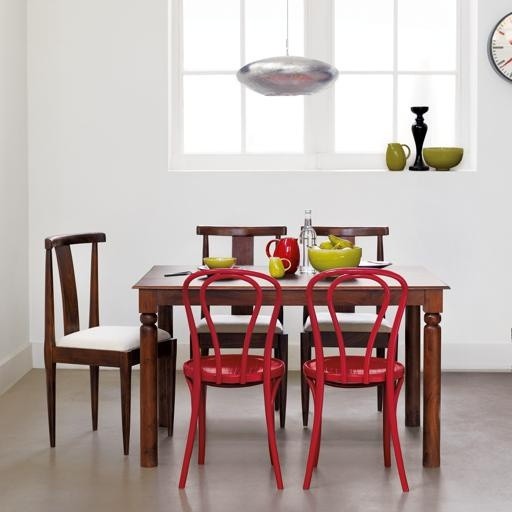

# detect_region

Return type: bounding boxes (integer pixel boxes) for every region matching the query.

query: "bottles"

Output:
[300,209,317,275]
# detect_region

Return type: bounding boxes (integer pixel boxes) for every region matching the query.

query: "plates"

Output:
[198,265,241,278]
[358,261,391,269]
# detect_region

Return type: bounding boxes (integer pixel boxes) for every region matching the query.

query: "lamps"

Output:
[236,0,338,97]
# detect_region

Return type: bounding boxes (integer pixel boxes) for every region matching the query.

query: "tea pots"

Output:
[386,143,411,171]
[269,257,291,279]
[265,237,300,275]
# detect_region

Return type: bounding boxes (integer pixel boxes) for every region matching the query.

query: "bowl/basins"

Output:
[205,257,236,269]
[423,148,464,171]
[308,248,362,281]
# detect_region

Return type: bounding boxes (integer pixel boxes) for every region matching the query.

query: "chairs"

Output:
[299,225,398,426]
[301,266,409,493]
[188,226,289,429]
[43,231,177,455]
[177,267,286,492]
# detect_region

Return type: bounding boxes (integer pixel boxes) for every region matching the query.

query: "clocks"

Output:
[487,12,512,85]
